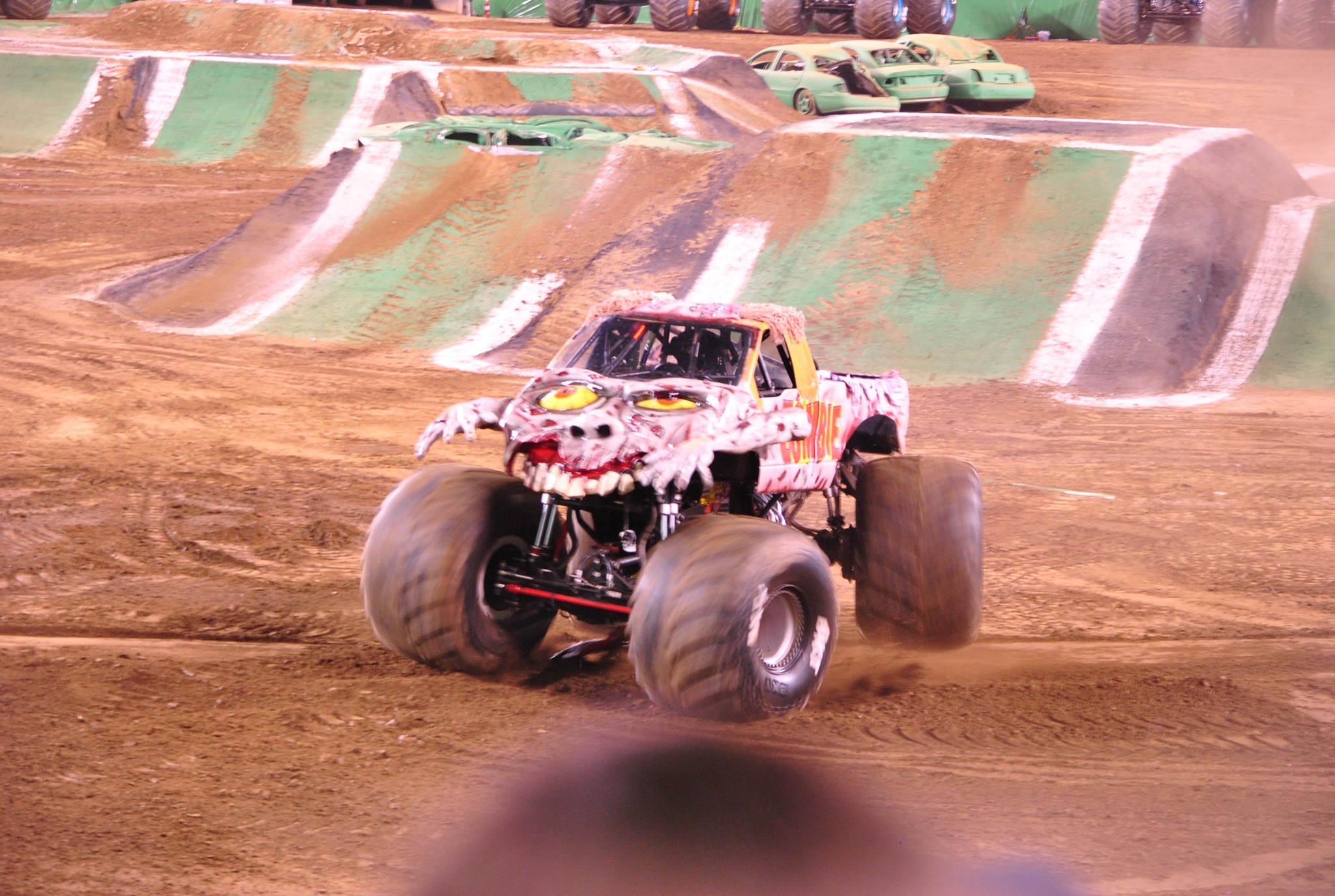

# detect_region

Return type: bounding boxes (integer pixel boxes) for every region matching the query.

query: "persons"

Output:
[662,329,726,376]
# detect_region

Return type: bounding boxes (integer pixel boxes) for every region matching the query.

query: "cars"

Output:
[356,115,620,149]
[894,33,1037,112]
[826,39,950,109]
[744,43,901,116]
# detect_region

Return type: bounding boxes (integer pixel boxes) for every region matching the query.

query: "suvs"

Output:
[362,286,988,731]
[544,0,958,40]
[1096,0,1334,51]
